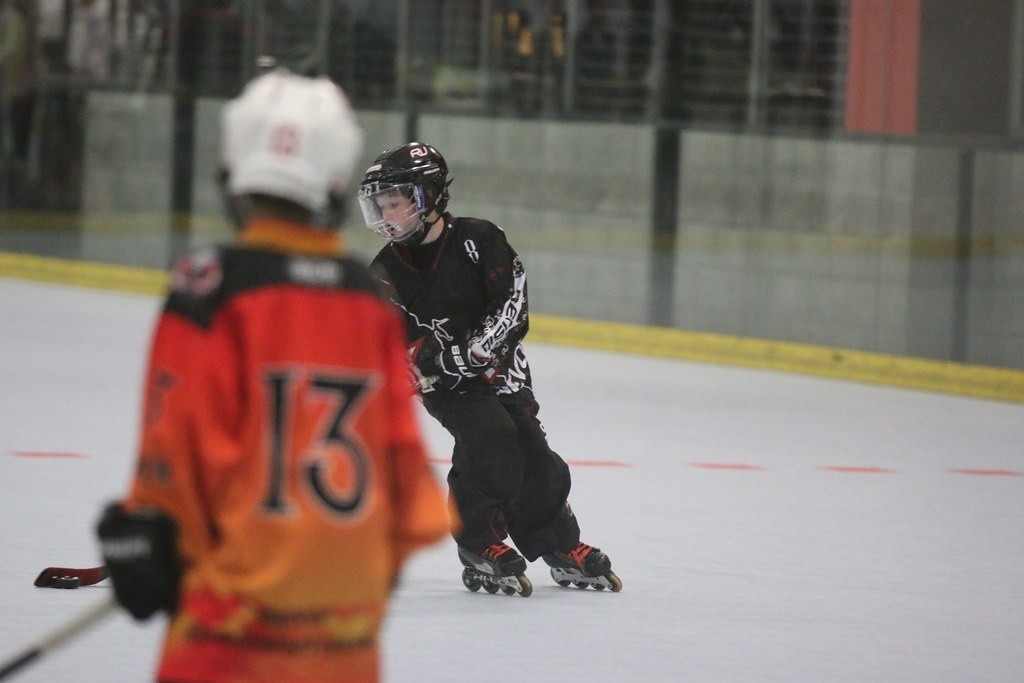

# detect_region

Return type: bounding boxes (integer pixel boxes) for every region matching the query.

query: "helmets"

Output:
[358,141,450,249]
[216,68,366,226]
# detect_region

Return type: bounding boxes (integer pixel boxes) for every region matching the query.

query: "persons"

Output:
[357,141,624,598]
[97,68,452,683]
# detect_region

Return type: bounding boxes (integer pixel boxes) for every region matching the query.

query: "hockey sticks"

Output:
[33,564,110,591]
[0,598,118,683]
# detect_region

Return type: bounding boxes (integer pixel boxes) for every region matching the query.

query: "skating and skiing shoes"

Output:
[457,535,532,597]
[541,538,621,592]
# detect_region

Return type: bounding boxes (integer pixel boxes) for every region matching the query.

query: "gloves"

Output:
[419,341,489,395]
[98,503,178,622]
